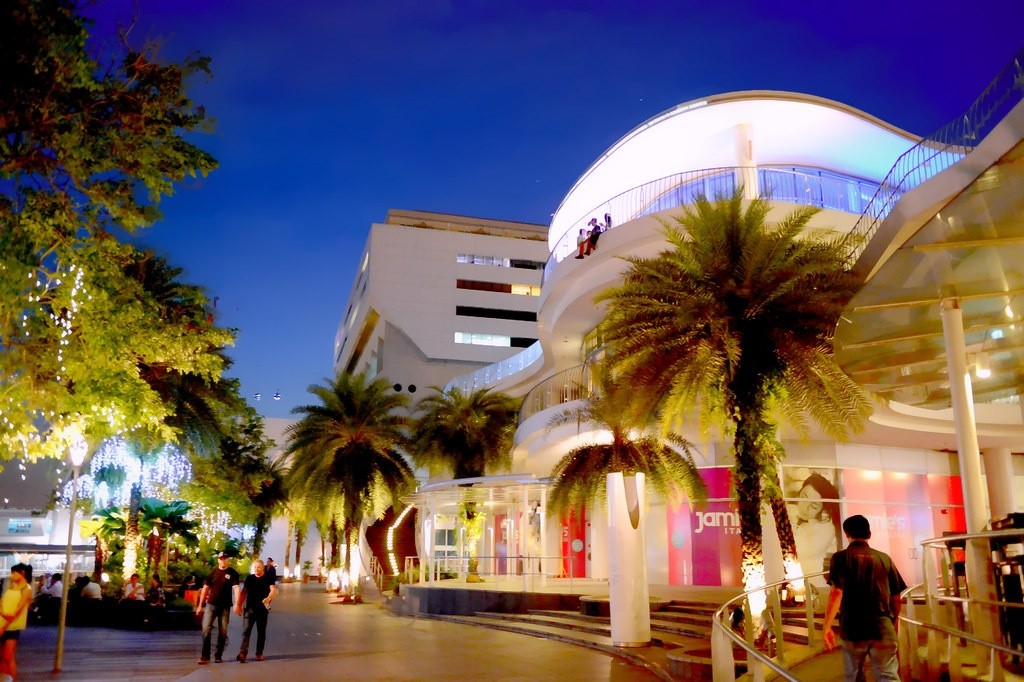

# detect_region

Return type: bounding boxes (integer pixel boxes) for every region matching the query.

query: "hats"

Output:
[218,551,229,557]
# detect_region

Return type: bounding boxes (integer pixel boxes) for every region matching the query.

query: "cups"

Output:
[264,602,272,610]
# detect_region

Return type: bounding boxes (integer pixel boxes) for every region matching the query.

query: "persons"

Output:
[0,551,276,682]
[822,515,907,682]
[574,213,613,259]
[786,474,840,588]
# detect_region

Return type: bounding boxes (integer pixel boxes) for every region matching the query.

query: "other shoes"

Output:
[236,654,246,663]
[198,656,209,664]
[215,658,222,663]
[256,655,263,660]
